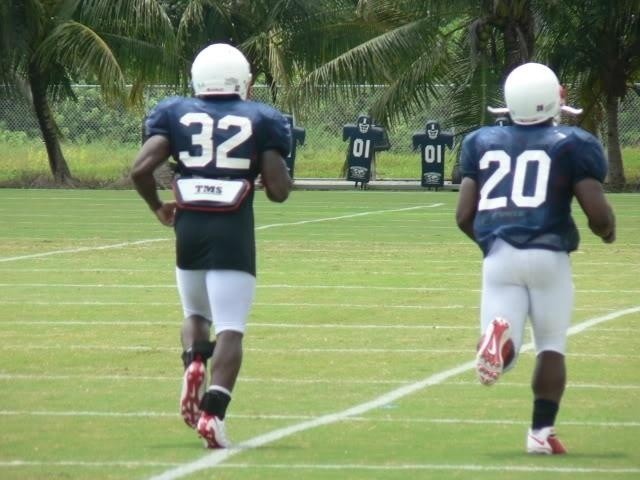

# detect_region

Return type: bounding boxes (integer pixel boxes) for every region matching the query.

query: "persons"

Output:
[130,42,292,448]
[455,61,617,453]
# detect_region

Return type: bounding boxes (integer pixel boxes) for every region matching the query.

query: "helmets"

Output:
[190,42,253,102]
[503,62,562,126]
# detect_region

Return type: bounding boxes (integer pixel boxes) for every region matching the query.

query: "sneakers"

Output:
[524,424,569,456]
[179,361,210,427]
[474,317,517,387]
[193,412,229,449]
